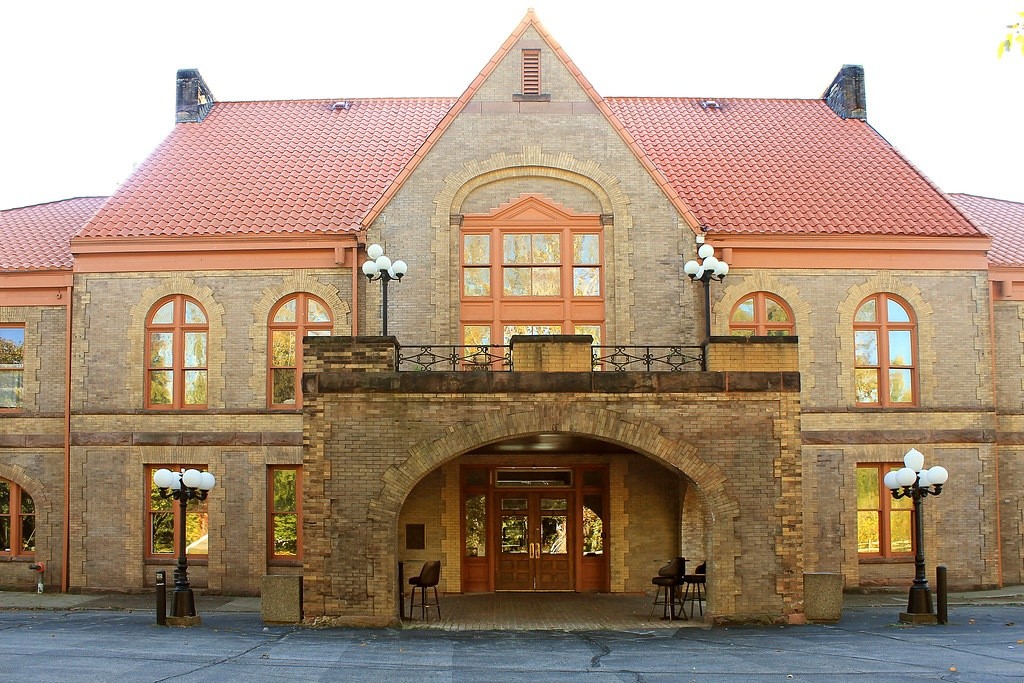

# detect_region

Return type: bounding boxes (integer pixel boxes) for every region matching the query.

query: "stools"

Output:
[647,556,689,622]
[677,559,707,618]
[408,560,443,621]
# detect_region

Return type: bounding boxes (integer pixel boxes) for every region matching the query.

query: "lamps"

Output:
[362,242,406,336]
[684,244,730,337]
